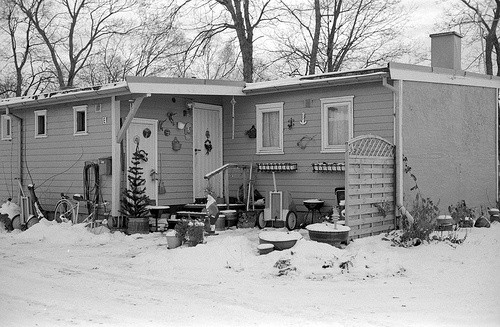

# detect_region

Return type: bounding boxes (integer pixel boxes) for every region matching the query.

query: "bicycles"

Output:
[53,193,112,235]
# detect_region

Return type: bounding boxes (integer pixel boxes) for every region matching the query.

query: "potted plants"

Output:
[117,142,152,234]
